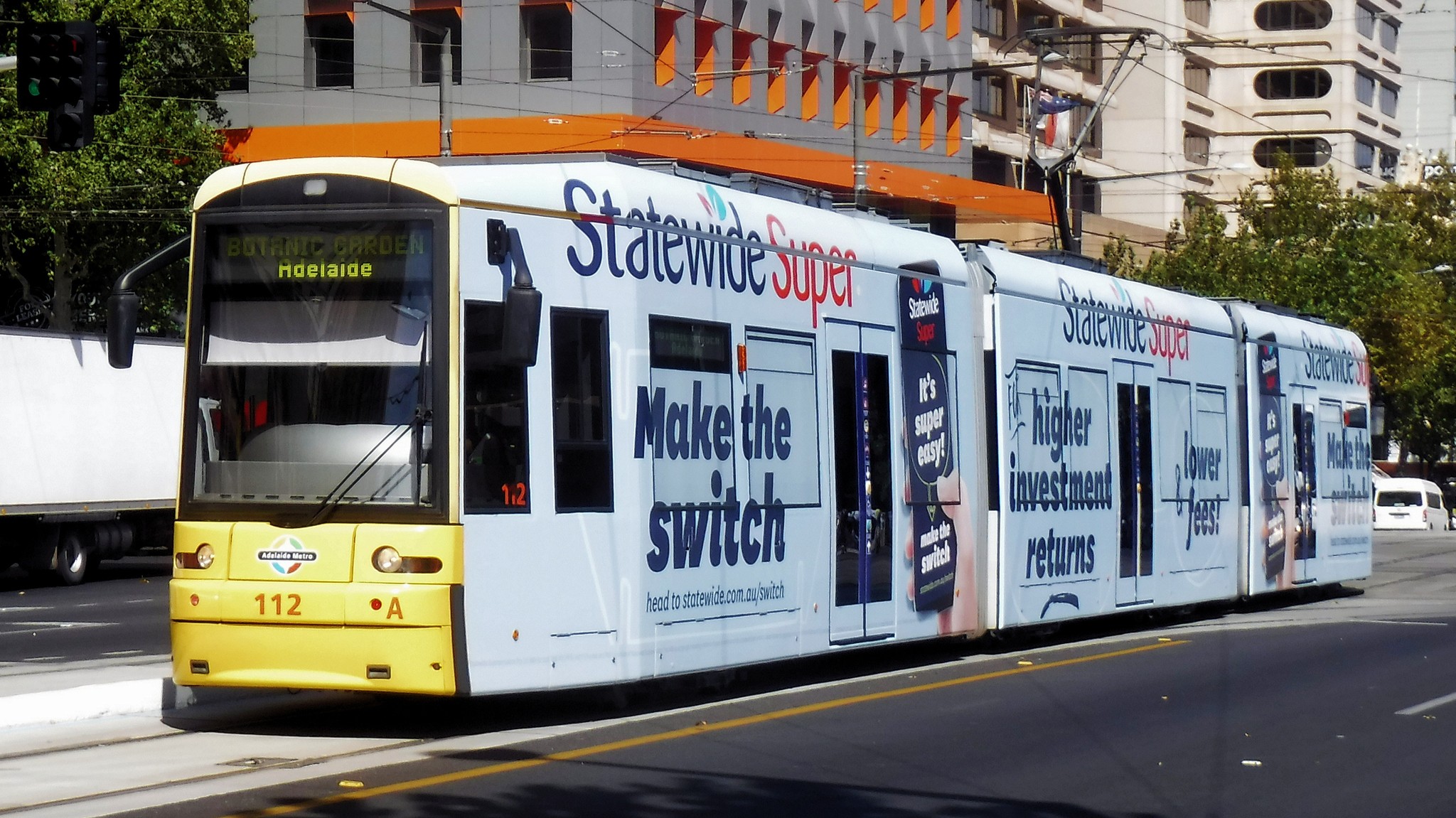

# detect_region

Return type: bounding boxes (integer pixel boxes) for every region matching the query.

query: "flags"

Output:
[1028,87,1079,159]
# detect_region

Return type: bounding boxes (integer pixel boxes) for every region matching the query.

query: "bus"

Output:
[106,24,1375,738]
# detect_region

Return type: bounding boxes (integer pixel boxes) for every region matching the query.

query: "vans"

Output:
[1371,477,1450,531]
